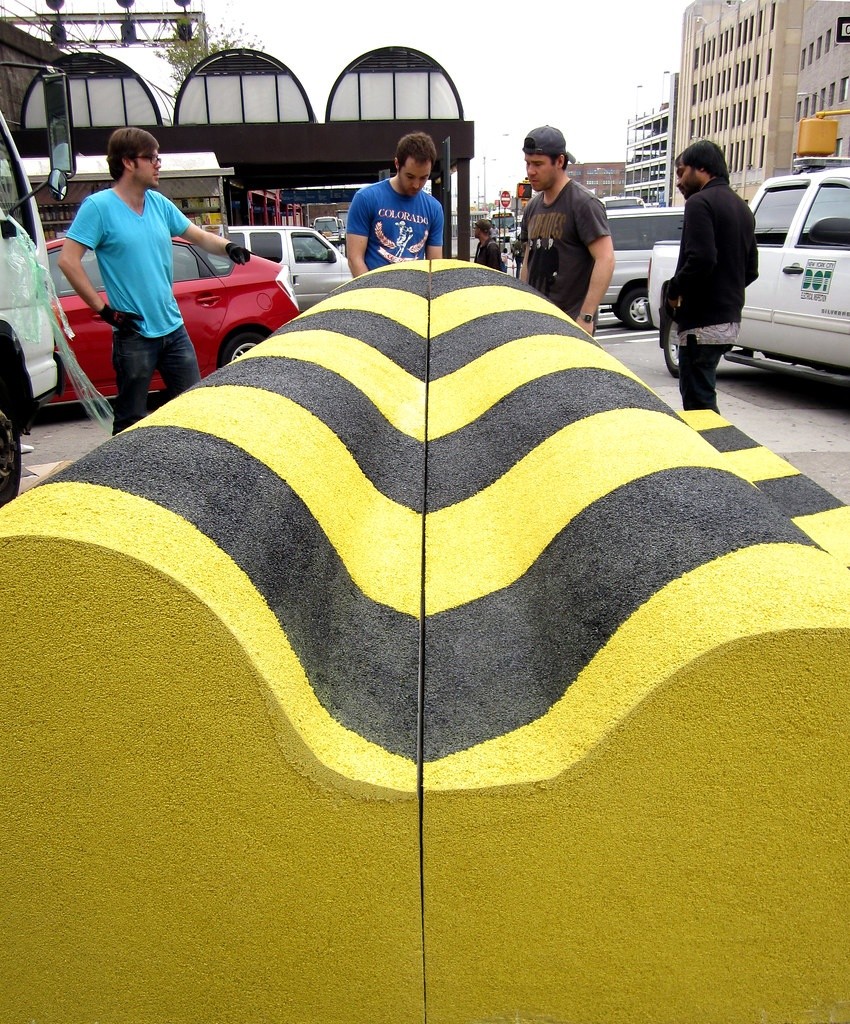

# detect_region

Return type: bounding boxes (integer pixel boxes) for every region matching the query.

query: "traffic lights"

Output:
[517,183,532,199]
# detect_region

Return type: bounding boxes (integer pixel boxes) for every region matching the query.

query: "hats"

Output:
[476,219,493,235]
[522,125,576,164]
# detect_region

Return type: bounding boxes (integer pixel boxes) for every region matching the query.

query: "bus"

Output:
[490,209,516,243]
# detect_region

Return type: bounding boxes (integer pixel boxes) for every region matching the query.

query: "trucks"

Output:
[310,216,341,241]
[0,60,76,508]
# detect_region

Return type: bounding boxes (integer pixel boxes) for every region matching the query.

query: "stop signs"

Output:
[501,191,511,208]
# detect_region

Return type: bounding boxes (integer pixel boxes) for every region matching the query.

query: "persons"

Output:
[500,248,513,273]
[489,224,500,241]
[474,219,501,271]
[346,133,444,278]
[666,139,759,415]
[511,234,526,279]
[520,125,614,336]
[57,128,251,436]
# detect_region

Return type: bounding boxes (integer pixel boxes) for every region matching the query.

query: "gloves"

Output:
[224,242,250,265]
[97,304,144,336]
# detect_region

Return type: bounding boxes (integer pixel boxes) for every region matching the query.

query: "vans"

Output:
[208,226,354,314]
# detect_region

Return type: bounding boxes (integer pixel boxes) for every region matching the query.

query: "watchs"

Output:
[578,311,596,324]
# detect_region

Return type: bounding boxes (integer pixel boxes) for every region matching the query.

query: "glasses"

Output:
[132,154,162,166]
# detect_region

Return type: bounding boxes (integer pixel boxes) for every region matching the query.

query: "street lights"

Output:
[477,176,479,210]
[797,92,830,111]
[483,156,486,204]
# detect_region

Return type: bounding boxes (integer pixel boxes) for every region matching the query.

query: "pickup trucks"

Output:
[647,156,850,389]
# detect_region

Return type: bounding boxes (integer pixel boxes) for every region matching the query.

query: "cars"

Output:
[597,206,685,331]
[40,236,300,408]
